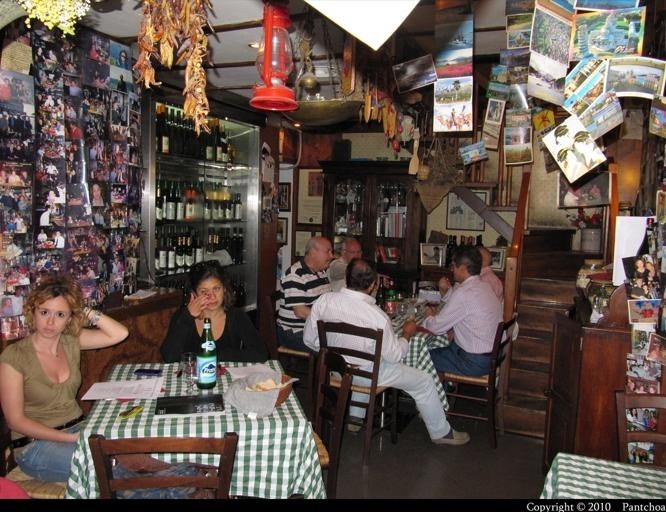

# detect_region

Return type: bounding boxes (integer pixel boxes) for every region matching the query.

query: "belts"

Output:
[7,414,85,448]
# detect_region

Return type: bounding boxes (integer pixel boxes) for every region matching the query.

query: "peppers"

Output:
[131,0,215,138]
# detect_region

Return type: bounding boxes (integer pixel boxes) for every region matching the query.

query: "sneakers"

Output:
[347,419,364,432]
[432,428,471,446]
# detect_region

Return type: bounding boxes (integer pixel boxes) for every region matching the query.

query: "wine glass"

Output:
[182,353,198,395]
[396,292,417,321]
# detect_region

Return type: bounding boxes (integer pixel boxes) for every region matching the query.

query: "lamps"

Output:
[248,0,299,112]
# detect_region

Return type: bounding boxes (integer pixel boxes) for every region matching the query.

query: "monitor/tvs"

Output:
[611,215,656,285]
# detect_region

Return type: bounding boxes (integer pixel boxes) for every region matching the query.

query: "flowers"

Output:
[565,206,603,228]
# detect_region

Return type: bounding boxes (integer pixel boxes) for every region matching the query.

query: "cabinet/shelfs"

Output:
[541,307,631,461]
[316,157,423,304]
[135,82,260,320]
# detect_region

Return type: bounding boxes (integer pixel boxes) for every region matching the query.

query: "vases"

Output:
[580,227,602,254]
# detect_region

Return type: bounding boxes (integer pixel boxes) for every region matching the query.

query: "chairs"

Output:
[0,275,521,498]
[613,390,666,473]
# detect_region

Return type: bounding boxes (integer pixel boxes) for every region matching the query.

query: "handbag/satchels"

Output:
[111,453,221,499]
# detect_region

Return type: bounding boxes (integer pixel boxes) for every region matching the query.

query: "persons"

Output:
[423,248,439,263]
[276,237,334,358]
[563,184,601,208]
[627,260,664,463]
[160,261,268,364]
[327,238,389,290]
[0,279,129,483]
[438,247,503,304]
[423,246,503,377]
[302,258,471,445]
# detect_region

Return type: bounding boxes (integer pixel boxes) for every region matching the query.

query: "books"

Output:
[376,206,404,239]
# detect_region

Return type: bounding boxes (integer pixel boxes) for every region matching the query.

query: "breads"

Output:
[246,379,276,391]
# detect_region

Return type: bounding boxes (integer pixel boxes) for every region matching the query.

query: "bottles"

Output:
[153,104,247,309]
[447,233,483,267]
[196,317,218,388]
[646,218,654,229]
[376,276,396,315]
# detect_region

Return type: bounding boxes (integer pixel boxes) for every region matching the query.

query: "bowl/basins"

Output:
[232,375,293,407]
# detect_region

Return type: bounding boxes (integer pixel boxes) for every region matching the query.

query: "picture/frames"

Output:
[277,216,289,246]
[419,242,448,269]
[279,182,291,213]
[485,245,507,272]
